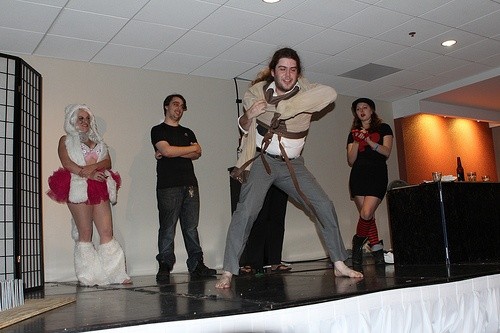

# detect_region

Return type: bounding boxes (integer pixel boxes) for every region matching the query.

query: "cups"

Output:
[481,176,490,182]
[432,172,442,183]
[467,172,476,181]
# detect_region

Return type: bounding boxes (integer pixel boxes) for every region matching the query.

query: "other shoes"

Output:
[155,261,170,280]
[190,260,219,276]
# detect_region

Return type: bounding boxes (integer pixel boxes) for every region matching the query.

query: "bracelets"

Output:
[373,144,378,150]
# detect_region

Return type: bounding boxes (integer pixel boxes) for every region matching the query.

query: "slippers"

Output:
[272,265,292,271]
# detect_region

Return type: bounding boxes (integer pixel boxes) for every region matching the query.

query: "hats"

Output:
[352,98,375,112]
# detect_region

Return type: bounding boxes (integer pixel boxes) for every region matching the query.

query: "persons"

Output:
[46,105,133,286]
[151,94,217,281]
[347,98,393,267]
[216,47,363,289]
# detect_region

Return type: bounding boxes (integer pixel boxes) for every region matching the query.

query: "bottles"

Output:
[457,157,464,182]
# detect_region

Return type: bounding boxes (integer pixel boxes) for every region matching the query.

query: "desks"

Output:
[388,181,500,265]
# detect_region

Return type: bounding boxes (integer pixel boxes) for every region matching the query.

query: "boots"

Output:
[353,234,368,265]
[367,239,385,265]
